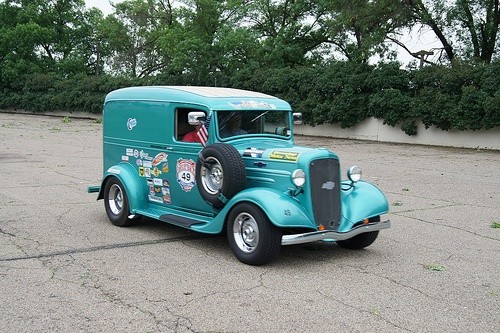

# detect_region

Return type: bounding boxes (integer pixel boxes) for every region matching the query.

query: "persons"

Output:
[183,112,208,144]
[221,113,247,138]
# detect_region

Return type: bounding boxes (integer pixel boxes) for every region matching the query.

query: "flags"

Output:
[197,110,213,145]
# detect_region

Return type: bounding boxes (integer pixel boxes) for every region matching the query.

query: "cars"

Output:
[88,85,391,266]
